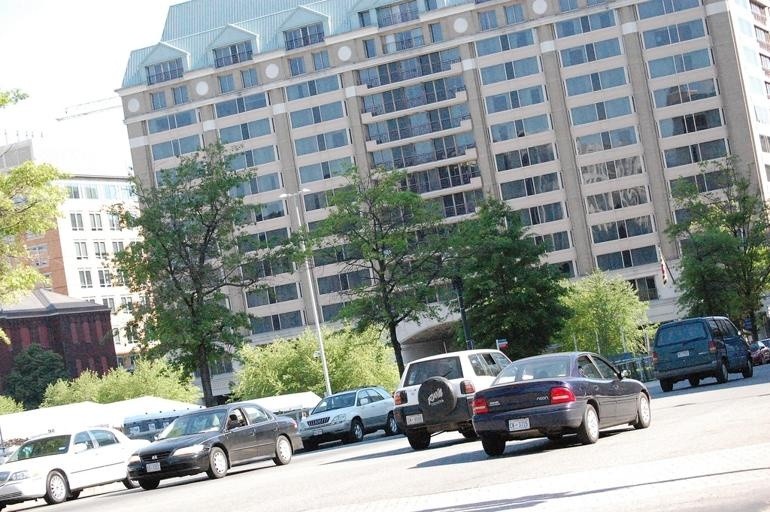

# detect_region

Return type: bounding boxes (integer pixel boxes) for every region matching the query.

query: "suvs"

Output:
[394,348,515,450]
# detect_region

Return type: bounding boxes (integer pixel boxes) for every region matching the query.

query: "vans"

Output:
[652,314,754,392]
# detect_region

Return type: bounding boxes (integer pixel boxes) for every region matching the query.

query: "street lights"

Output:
[277,187,331,394]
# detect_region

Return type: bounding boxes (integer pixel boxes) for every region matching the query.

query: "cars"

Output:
[750,340,770,366]
[1,425,153,505]
[471,350,653,456]
[127,400,299,491]
[298,385,398,450]
